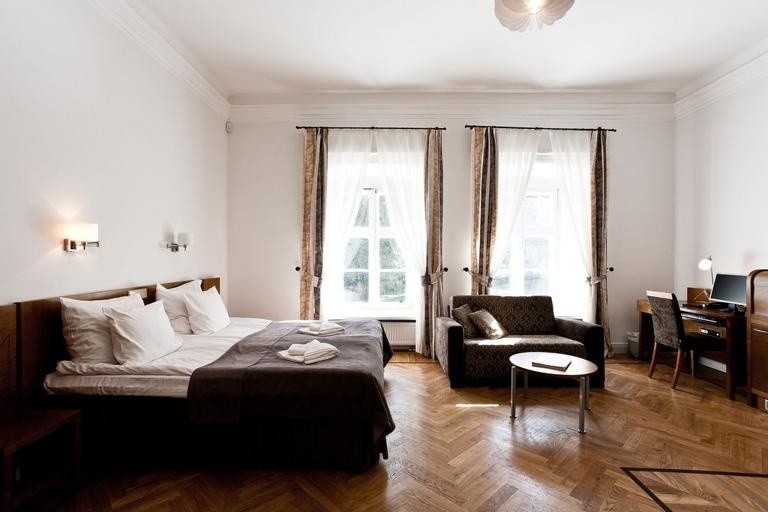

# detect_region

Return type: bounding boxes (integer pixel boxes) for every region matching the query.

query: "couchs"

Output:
[448,297,604,389]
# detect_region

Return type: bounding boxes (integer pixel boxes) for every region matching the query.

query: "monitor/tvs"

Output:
[709,273,748,314]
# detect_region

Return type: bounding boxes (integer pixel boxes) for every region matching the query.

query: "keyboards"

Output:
[681,312,718,323]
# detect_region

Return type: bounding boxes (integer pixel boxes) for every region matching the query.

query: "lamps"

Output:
[698,255,714,286]
[166,232,190,256]
[64,223,104,252]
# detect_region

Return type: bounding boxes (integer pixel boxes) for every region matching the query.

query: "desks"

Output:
[637,297,746,400]
[509,351,597,435]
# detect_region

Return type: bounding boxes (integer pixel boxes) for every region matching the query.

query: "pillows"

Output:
[467,308,509,339]
[59,279,231,366]
[454,304,485,338]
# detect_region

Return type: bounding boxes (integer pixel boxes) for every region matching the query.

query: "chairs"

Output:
[646,290,700,390]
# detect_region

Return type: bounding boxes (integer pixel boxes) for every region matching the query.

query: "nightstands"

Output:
[0,406,85,511]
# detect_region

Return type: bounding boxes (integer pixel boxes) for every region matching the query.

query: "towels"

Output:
[276,321,344,365]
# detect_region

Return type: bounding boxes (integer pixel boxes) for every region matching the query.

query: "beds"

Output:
[43,317,382,473]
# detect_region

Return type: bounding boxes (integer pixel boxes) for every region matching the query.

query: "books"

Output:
[532,356,573,371]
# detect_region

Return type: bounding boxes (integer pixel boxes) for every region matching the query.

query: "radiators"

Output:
[380,320,416,352]
